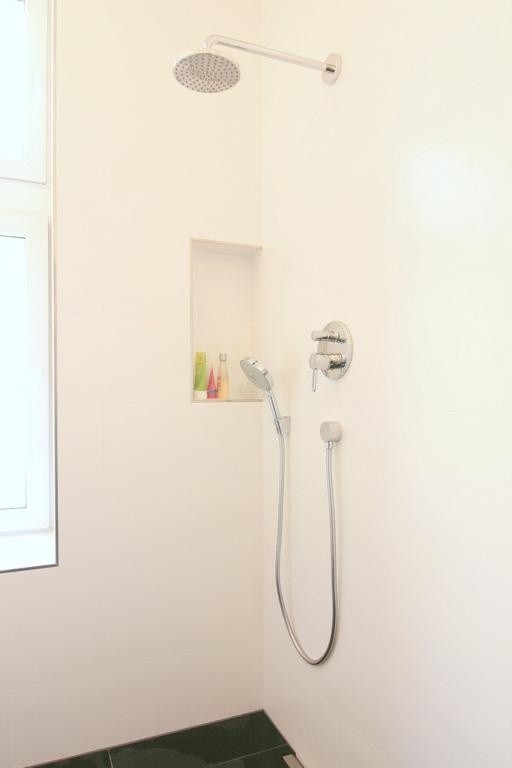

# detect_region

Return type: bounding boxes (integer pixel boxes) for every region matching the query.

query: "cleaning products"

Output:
[192,350,210,401]
[216,353,230,402]
[206,367,217,401]
